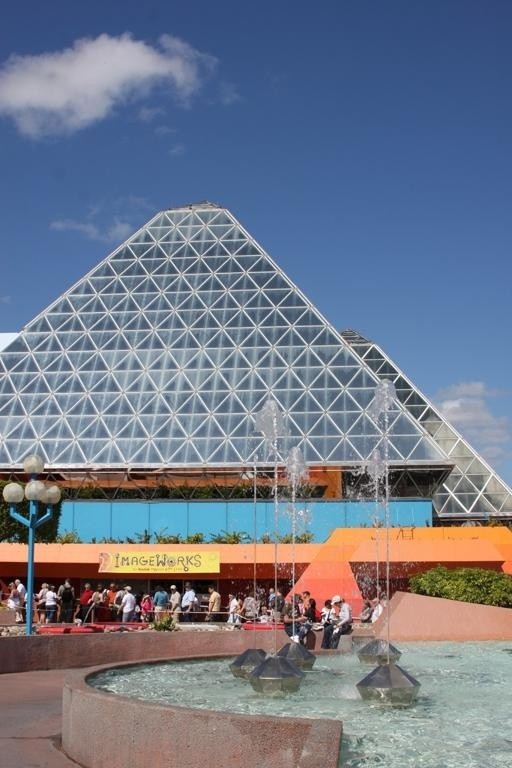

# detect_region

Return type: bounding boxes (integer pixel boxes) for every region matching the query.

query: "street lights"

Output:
[2,453,63,636]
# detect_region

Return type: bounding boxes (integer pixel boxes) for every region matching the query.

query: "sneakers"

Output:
[290,635,300,644]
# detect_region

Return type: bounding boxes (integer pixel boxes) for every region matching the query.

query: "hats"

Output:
[302,591,310,595]
[331,596,341,605]
[124,586,131,591]
[183,582,193,587]
[171,586,175,590]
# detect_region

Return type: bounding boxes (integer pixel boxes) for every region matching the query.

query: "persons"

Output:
[8,579,382,650]
